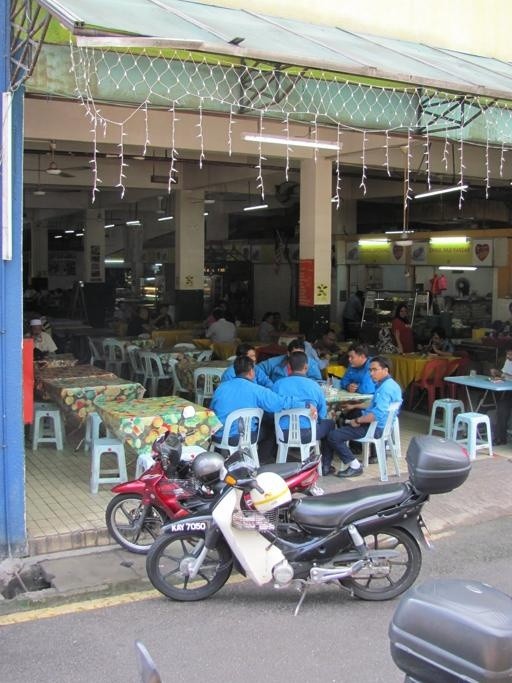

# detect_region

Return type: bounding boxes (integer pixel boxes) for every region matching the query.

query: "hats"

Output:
[30,319,41,326]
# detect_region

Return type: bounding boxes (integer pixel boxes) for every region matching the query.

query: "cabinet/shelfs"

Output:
[360,290,430,329]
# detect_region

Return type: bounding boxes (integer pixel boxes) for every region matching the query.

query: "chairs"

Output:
[138,351,170,396]
[87,336,101,365]
[336,402,401,483]
[171,358,190,397]
[193,367,223,406]
[102,341,125,374]
[127,345,146,382]
[195,349,213,362]
[447,359,469,399]
[211,407,264,469]
[408,360,448,410]
[274,407,322,479]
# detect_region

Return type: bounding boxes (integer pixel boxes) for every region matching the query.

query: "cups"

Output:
[334,380,340,389]
[470,370,476,379]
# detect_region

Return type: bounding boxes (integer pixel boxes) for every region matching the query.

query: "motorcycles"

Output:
[145,416,473,616]
[105,404,325,555]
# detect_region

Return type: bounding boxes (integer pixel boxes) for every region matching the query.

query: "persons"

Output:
[202,305,224,328]
[391,303,414,354]
[126,304,172,337]
[417,326,454,357]
[326,356,403,478]
[313,328,345,359]
[489,341,512,446]
[273,350,337,476]
[208,356,318,446]
[219,300,235,323]
[338,342,377,455]
[272,311,288,343]
[342,290,364,339]
[256,339,306,385]
[205,309,238,343]
[219,343,274,388]
[302,327,331,380]
[25,311,59,355]
[257,312,286,342]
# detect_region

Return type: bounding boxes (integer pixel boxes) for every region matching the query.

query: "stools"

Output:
[451,411,493,461]
[89,437,128,494]
[84,413,102,451]
[429,398,464,440]
[32,407,66,451]
[180,446,207,462]
[135,454,156,480]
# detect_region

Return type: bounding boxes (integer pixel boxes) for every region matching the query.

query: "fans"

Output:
[22,140,90,178]
[23,152,82,195]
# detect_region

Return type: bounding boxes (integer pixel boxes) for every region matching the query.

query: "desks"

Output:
[152,327,258,349]
[192,338,241,349]
[38,364,113,380]
[382,353,462,391]
[44,379,146,452]
[321,361,348,379]
[322,384,372,404]
[443,375,512,439]
[94,396,224,454]
[195,360,227,382]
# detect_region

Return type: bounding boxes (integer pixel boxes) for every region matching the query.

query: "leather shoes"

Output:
[492,437,507,446]
[368,456,378,464]
[322,466,335,476]
[337,464,364,477]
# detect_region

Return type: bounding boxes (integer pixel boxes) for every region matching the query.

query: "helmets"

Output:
[191,452,226,486]
[249,471,292,514]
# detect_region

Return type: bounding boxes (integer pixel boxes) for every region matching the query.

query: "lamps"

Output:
[158,217,173,221]
